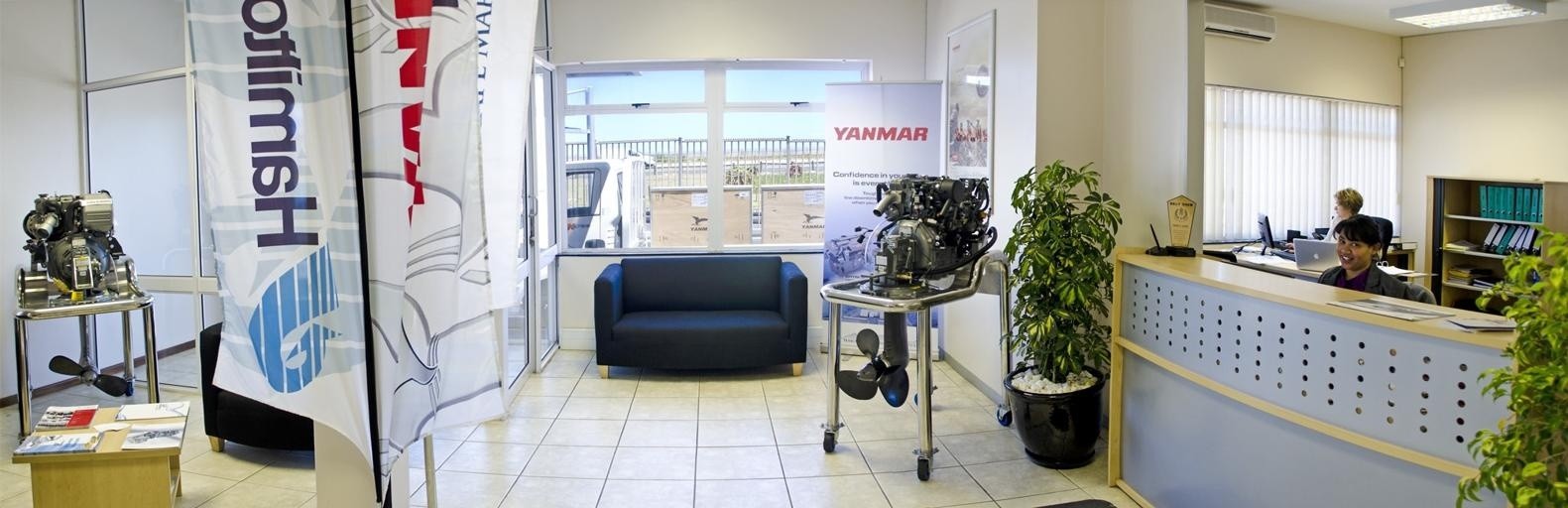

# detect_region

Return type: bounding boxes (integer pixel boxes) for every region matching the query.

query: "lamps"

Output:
[1390,2,1548,32]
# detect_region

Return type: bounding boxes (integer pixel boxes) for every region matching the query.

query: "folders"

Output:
[1479,185,1543,256]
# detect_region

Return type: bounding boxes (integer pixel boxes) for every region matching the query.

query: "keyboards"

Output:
[1270,250,1295,262]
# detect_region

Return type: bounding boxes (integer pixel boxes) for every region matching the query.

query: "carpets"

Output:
[1033,497,1117,508]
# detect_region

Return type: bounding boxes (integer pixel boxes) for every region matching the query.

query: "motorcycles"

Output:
[786,162,801,177]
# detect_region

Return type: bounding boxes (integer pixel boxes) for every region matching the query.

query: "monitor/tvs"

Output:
[1257,211,1275,255]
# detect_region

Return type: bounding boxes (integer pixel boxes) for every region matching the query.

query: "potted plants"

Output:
[996,157,1128,473]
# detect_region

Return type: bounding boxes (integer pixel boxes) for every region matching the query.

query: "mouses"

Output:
[1283,248,1290,252]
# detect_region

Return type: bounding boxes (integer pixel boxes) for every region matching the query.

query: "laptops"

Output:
[1293,237,1340,272]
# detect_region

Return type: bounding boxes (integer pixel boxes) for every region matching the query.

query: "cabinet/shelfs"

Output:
[1424,171,1568,319]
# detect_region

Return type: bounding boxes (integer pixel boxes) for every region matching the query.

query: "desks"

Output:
[8,399,194,508]
[1231,243,1418,285]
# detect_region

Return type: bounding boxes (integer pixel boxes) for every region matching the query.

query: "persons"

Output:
[1318,214,1416,301]
[950,103,982,166]
[1285,188,1363,254]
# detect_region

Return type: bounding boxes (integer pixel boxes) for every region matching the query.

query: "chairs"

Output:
[198,321,314,455]
[1369,215,1395,263]
[1403,280,1438,307]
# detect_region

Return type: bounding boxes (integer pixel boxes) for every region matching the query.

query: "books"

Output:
[37,404,99,430]
[1448,316,1518,331]
[13,433,104,455]
[1446,240,1505,289]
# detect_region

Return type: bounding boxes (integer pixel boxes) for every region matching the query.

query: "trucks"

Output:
[606,146,655,169]
[566,152,828,337]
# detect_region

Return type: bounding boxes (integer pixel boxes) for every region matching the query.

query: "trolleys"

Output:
[814,248,1018,480]
[14,291,162,450]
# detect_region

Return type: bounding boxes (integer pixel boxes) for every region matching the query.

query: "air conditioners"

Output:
[1205,2,1278,47]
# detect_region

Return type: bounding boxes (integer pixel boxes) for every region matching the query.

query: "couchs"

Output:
[591,251,810,384]
